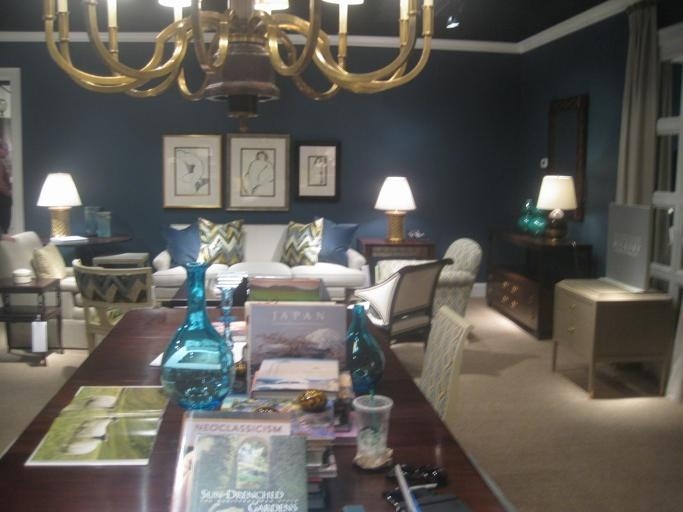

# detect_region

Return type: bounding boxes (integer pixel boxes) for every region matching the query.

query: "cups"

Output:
[353,395,393,469]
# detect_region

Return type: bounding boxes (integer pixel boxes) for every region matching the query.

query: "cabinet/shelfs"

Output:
[551,278,674,398]
[486,232,594,340]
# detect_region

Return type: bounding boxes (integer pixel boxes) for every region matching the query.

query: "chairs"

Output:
[72,258,161,354]
[417,306,475,425]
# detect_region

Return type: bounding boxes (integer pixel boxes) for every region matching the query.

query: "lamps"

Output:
[445,14,461,30]
[36,172,83,239]
[40,0,438,133]
[374,176,417,242]
[536,174,578,238]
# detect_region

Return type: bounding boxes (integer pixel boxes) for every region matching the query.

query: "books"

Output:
[172,395,337,506]
[242,299,361,448]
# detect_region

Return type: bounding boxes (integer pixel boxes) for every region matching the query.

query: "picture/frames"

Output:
[162,132,222,209]
[225,132,291,212]
[293,140,341,202]
[548,93,588,222]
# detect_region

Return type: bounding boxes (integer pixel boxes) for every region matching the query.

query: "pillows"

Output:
[312,214,360,267]
[34,242,67,280]
[280,217,325,267]
[196,216,244,266]
[163,222,200,268]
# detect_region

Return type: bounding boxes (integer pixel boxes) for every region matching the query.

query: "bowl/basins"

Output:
[11,268,32,284]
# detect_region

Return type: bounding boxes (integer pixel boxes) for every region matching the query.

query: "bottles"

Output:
[94,211,112,236]
[85,206,104,236]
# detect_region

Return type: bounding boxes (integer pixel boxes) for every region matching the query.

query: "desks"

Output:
[41,231,133,267]
[356,236,436,260]
[92,252,149,267]
[0,305,507,512]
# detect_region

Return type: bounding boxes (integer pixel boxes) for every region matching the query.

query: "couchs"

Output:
[150,223,371,307]
[374,237,483,333]
[354,257,453,349]
[0,230,97,350]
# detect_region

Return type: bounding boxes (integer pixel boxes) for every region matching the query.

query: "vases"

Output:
[347,304,386,393]
[159,261,236,410]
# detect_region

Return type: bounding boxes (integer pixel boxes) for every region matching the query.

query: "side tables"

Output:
[0,276,64,367]
